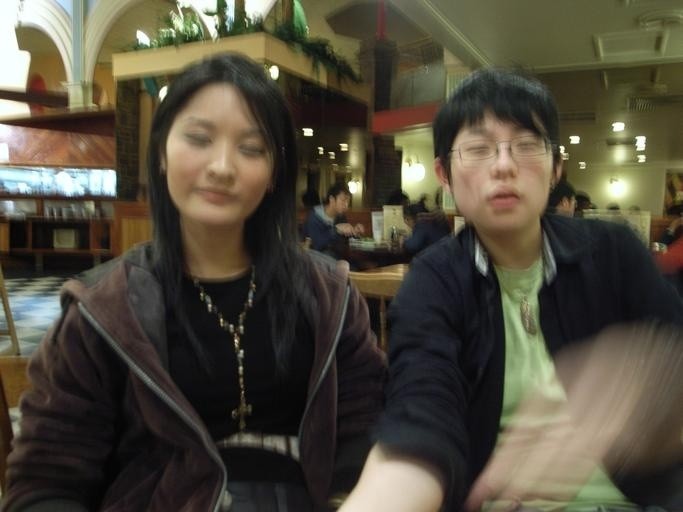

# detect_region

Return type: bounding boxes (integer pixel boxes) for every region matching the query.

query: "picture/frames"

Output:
[48,225,81,249]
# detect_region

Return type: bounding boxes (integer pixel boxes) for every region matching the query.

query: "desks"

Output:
[349,242,408,252]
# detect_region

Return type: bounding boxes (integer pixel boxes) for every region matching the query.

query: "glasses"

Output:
[446,135,555,164]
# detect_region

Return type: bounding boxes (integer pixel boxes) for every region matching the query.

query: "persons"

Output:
[4,50,388,512]
[297,182,364,269]
[547,178,578,217]
[334,65,682,512]
[656,204,682,248]
[397,194,451,258]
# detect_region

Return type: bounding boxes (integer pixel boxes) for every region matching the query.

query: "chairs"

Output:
[347,272,411,352]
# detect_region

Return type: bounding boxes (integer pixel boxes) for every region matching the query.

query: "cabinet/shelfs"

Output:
[0,190,117,270]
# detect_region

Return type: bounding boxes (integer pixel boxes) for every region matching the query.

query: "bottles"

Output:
[390,225,400,254]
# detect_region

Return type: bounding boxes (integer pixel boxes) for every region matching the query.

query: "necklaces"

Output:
[183,262,258,436]
[493,246,543,335]
